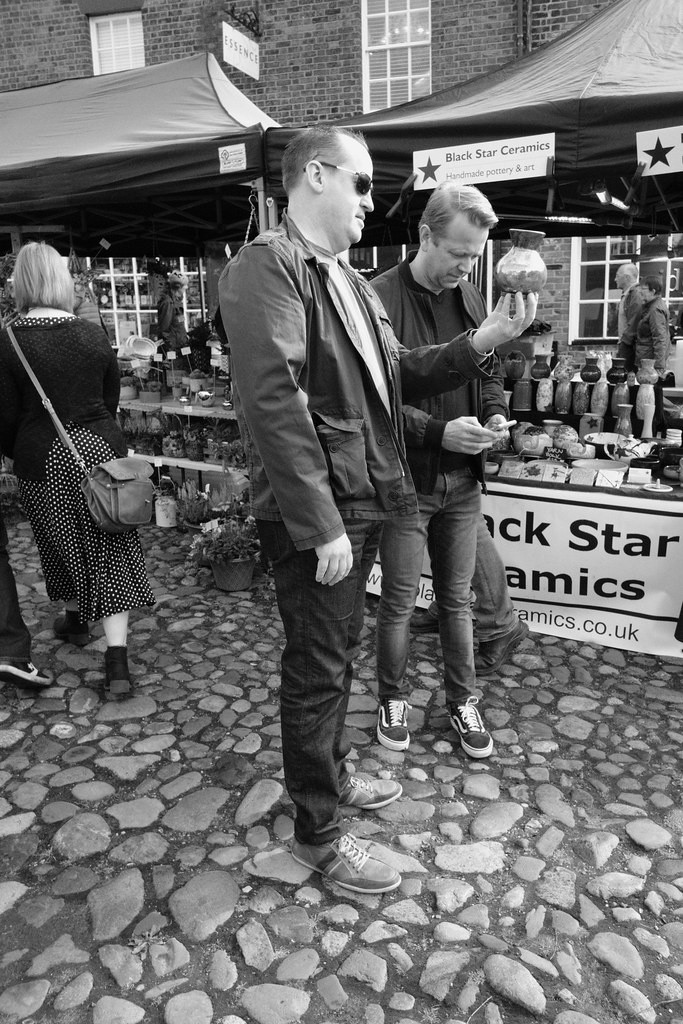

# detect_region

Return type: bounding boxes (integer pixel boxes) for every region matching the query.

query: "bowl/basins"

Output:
[485,462,499,474]
[583,431,627,457]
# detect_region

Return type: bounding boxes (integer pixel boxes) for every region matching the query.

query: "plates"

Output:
[573,458,628,475]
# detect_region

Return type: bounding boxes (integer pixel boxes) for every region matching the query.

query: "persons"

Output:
[609,261,671,380]
[215,306,230,355]
[219,123,539,893]
[149,270,190,351]
[365,184,529,758]
[0,243,156,693]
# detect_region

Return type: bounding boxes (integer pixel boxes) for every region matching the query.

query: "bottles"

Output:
[497,227,549,315]
[484,350,683,460]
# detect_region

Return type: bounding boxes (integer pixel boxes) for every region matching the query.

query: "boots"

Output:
[103,646,132,693]
[53,610,89,645]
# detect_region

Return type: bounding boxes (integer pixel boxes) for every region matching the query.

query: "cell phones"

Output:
[489,419,517,432]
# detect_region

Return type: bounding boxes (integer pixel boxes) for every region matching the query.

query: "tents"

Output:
[0,52,279,325]
[263,0,683,236]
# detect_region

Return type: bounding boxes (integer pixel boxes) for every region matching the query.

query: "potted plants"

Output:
[100,364,264,590]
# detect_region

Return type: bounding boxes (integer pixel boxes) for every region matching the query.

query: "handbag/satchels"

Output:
[81,457,154,533]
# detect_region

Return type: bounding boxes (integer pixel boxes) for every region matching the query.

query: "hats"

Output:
[170,272,188,289]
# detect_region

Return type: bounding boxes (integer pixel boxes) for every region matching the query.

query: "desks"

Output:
[366,378,683,663]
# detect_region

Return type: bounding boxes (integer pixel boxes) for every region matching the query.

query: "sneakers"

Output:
[376,699,412,751]
[409,610,438,633]
[474,620,529,674]
[450,696,493,758]
[0,661,50,688]
[291,832,401,893]
[338,775,402,809]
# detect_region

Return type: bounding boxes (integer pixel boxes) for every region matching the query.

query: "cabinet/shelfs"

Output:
[105,371,265,523]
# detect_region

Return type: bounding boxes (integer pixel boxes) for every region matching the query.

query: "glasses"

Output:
[303,162,374,197]
